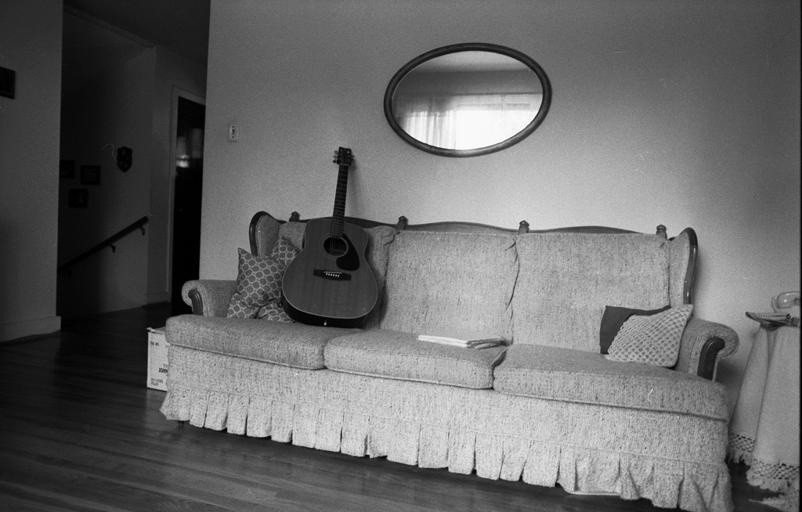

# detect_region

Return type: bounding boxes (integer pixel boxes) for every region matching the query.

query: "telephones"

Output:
[770,289,801,317]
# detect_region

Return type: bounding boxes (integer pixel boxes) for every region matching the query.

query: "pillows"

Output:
[598,303,694,369]
[225,237,302,324]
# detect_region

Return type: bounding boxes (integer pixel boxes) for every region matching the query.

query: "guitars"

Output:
[282,147,379,328]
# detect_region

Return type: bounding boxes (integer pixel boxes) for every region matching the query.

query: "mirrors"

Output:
[383,41,551,159]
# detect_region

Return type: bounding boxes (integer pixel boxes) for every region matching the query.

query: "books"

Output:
[418,331,503,349]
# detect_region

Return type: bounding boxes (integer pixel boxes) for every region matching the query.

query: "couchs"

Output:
[163,210,740,512]
